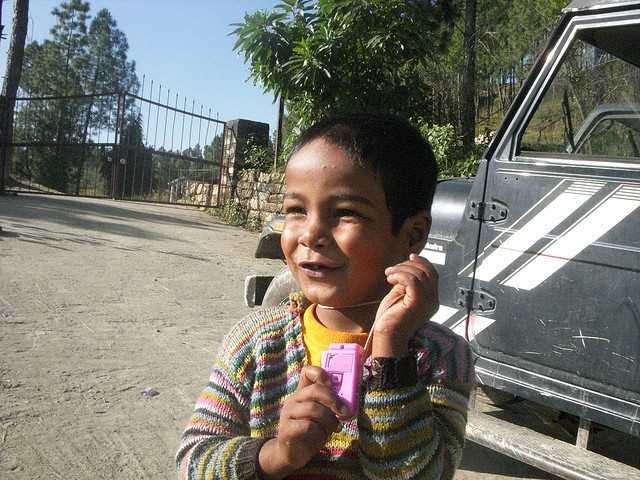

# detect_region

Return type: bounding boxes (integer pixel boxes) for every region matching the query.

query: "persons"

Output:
[174,106,475,479]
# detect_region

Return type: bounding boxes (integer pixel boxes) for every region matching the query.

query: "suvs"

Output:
[245,1,640,479]
